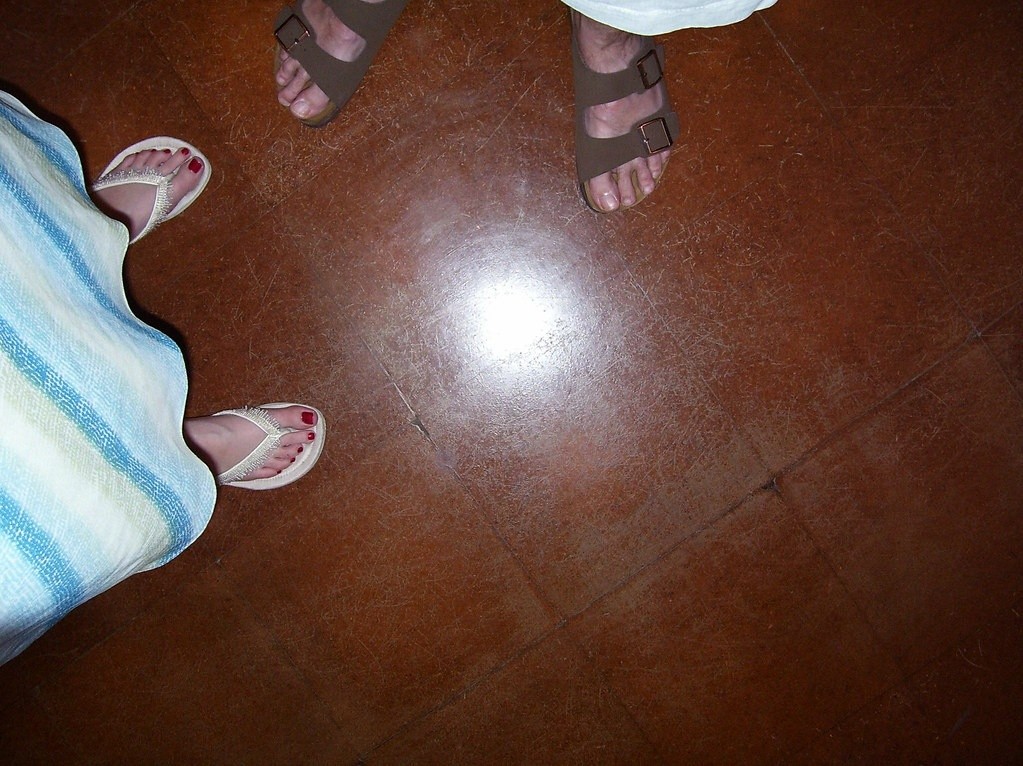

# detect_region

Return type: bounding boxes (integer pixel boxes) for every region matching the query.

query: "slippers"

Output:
[187,403,326,493]
[566,5,680,214]
[271,1,409,127]
[86,135,212,247]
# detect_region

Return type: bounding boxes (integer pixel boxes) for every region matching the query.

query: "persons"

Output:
[0,90,326,668]
[273,0,781,214]
